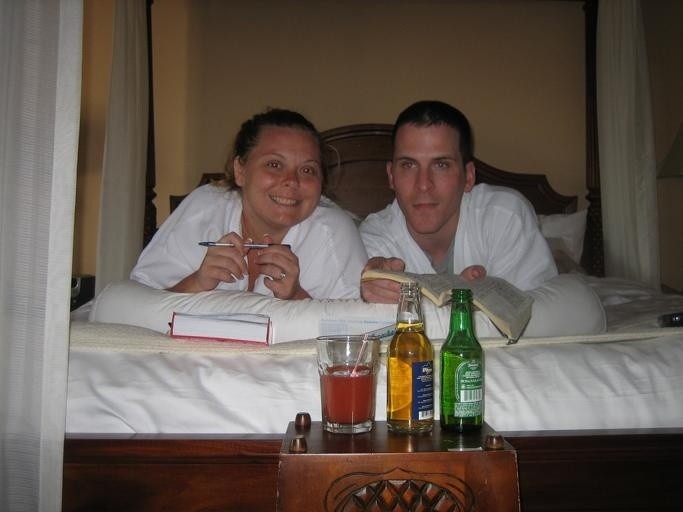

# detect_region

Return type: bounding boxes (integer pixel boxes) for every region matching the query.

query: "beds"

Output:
[19,121,681,504]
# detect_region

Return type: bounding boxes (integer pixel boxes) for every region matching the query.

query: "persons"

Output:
[128,105,369,299]
[358,100,559,306]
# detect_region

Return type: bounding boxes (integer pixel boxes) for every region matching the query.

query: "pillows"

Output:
[535,209,588,268]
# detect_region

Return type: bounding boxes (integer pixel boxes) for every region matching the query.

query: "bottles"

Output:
[657,312,683,329]
[386,281,432,435]
[439,285,487,439]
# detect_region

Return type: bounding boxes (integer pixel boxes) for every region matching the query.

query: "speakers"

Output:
[70,274,95,311]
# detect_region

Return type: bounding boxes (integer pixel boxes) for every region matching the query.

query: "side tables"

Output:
[278,414,520,511]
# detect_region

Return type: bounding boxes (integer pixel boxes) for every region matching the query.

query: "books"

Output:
[170,311,270,346]
[362,268,533,343]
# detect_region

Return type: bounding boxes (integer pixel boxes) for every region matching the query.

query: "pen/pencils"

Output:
[199,241,291,249]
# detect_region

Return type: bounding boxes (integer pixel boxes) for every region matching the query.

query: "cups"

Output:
[314,332,383,437]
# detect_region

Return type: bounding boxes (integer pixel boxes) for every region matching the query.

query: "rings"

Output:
[279,271,287,280]
